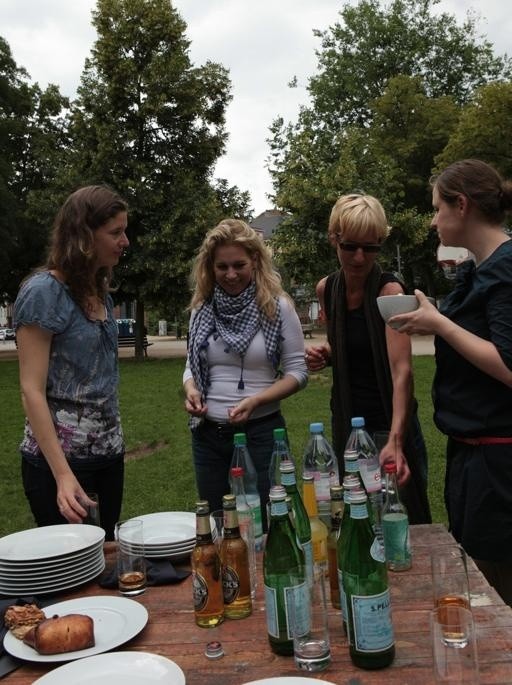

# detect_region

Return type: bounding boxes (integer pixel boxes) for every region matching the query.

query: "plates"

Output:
[240,674,335,684]
[0,523,106,598]
[32,650,186,684]
[3,596,149,662]
[119,511,219,562]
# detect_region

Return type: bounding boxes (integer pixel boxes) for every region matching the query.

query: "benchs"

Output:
[301,323,314,339]
[117,335,152,357]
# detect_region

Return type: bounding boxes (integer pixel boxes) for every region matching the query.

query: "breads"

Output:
[4,603,95,655]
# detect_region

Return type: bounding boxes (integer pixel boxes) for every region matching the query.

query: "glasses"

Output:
[335,232,383,253]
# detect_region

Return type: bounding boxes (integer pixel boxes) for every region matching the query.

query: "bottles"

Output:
[276,459,320,608]
[192,433,263,630]
[261,484,312,656]
[330,413,413,674]
[305,423,341,515]
[268,426,297,484]
[296,472,335,580]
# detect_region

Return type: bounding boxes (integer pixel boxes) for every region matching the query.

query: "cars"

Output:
[0,327,16,340]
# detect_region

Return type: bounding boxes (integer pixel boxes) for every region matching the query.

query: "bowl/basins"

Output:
[374,294,441,333]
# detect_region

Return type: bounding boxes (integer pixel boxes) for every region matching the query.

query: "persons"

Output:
[182,217,309,513]
[387,157,512,605]
[303,194,431,525]
[13,186,130,541]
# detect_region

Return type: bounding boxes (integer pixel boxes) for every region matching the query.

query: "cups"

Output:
[430,605,479,684]
[72,493,101,528]
[430,544,472,648]
[114,519,147,594]
[285,565,333,670]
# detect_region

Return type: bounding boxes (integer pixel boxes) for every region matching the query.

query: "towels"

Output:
[99,553,192,589]
[0,596,44,678]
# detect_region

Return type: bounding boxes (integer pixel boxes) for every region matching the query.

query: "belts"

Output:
[203,420,233,441]
[450,436,512,446]
